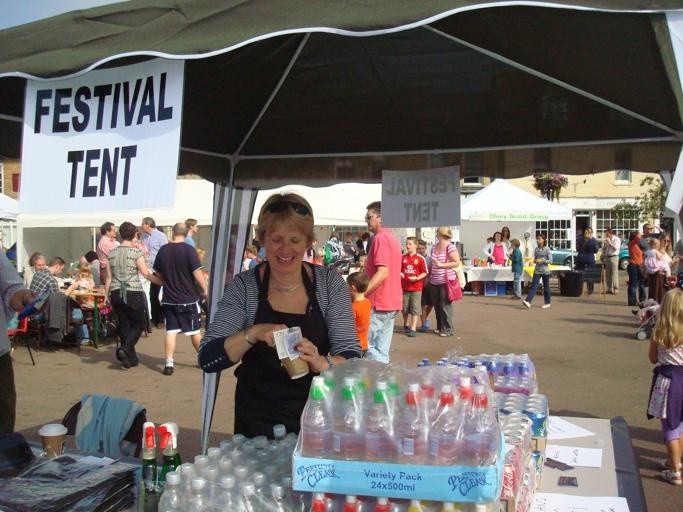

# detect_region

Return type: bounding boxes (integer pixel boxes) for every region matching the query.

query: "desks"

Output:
[460,265,571,298]
[0,442,161,512]
[520,415,645,512]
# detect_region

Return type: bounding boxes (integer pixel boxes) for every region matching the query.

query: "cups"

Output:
[273,342,309,380]
[39,424,67,457]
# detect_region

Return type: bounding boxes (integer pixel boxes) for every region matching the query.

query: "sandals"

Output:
[662,458,682,470]
[661,470,682,486]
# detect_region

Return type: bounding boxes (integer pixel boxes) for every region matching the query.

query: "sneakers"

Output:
[164,367,173,375]
[522,300,530,308]
[542,304,550,309]
[404,320,454,337]
[116,347,138,368]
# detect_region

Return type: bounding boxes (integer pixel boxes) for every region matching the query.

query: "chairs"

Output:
[329,260,352,275]
[7,287,148,366]
[63,395,146,458]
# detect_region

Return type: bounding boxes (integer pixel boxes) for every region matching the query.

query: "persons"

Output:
[0,243,47,440]
[344,272,372,357]
[359,201,402,364]
[241,202,460,337]
[153,224,208,375]
[0,217,208,345]
[102,222,165,368]
[484,227,621,309]
[627,223,683,486]
[199,193,364,438]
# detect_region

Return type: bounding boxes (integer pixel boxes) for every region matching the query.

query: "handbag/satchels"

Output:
[447,278,462,302]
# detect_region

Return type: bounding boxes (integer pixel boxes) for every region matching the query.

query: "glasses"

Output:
[262,201,311,215]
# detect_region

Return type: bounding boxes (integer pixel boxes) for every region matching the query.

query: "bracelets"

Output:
[244,327,255,346]
[323,353,333,368]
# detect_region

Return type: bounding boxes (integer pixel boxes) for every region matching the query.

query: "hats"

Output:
[437,225,452,238]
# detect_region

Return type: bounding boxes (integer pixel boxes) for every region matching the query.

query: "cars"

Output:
[548,245,578,267]
[600,239,631,270]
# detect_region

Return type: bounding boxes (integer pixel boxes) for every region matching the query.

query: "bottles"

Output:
[474,255,487,267]
[159,353,538,511]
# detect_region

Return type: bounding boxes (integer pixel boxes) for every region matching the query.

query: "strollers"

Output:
[633,299,659,341]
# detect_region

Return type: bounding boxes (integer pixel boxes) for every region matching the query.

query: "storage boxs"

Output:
[497,281,506,297]
[484,280,497,297]
